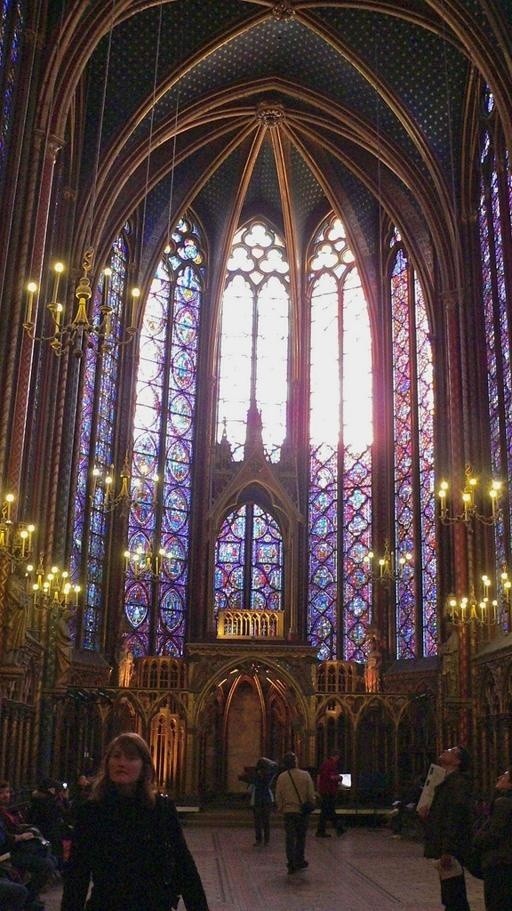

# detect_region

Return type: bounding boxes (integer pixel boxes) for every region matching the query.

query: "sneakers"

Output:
[288,861,308,874]
[315,831,331,838]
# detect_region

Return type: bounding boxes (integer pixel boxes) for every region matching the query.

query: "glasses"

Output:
[448,748,460,760]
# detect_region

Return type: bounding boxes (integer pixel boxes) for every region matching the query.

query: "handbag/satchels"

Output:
[302,800,315,813]
[250,786,255,806]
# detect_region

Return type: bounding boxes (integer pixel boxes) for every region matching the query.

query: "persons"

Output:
[420,745,472,911]
[2,767,101,911]
[63,731,209,911]
[313,751,345,839]
[387,774,426,840]
[273,753,320,875]
[476,768,511,911]
[238,757,278,846]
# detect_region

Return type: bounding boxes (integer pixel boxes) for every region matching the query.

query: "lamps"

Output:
[23,0,140,355]
[31,0,80,619]
[450,0,498,627]
[89,0,157,528]
[120,0,167,584]
[1,0,36,578]
[502,1,511,605]
[363,0,412,587]
[438,0,502,533]
